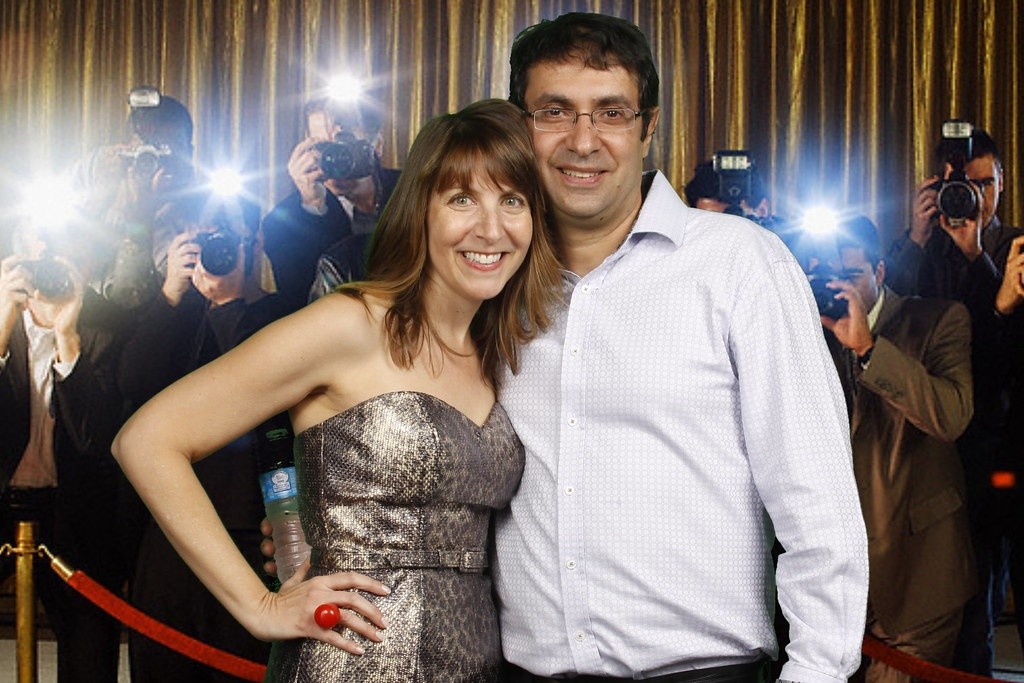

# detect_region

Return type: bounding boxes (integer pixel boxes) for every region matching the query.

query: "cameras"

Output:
[712,149,792,246]
[922,117,986,226]
[800,204,855,317]
[184,171,257,276]
[15,249,71,298]
[305,73,378,181]
[117,86,173,182]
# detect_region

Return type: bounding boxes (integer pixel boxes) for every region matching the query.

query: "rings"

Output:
[314,603,341,629]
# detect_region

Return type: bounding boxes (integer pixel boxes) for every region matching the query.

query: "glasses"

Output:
[524,108,649,132]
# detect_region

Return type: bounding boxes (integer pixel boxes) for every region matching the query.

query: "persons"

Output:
[684,121,1024,680]
[0,85,404,683]
[111,99,569,681]
[258,13,872,683]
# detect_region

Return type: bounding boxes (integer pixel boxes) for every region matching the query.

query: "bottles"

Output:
[258,429,312,584]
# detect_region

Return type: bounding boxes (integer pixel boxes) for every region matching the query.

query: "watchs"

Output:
[859,334,879,370]
